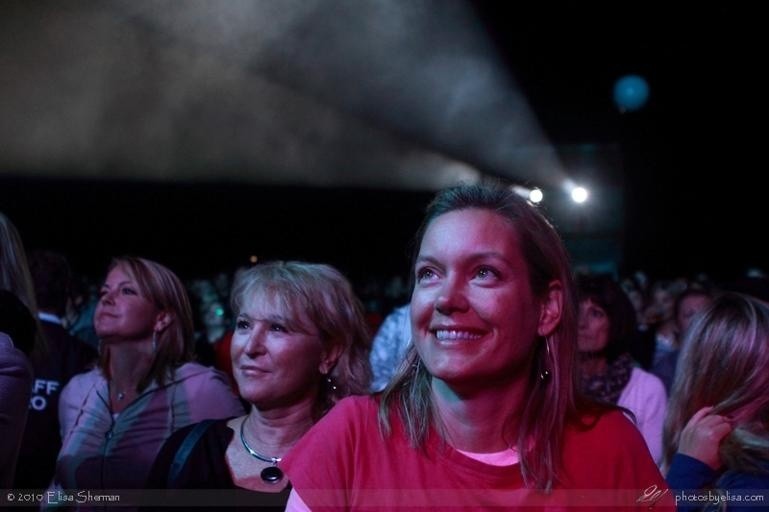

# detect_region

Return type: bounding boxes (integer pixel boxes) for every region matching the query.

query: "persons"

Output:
[0,180,768,512]
